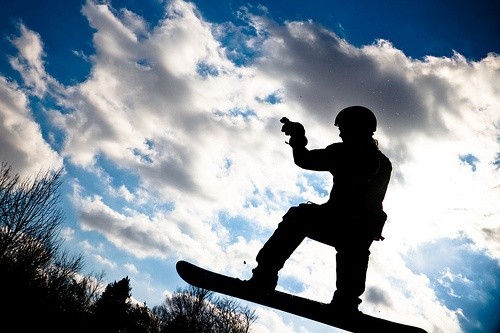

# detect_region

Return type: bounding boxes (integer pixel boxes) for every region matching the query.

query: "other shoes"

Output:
[245,268,279,290]
[327,291,359,312]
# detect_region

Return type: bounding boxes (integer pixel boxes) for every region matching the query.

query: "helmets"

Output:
[333,104,379,142]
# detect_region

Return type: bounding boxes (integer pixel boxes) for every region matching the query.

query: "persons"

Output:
[241,105,393,314]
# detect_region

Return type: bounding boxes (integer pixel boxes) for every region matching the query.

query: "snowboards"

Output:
[169,258,427,333]
